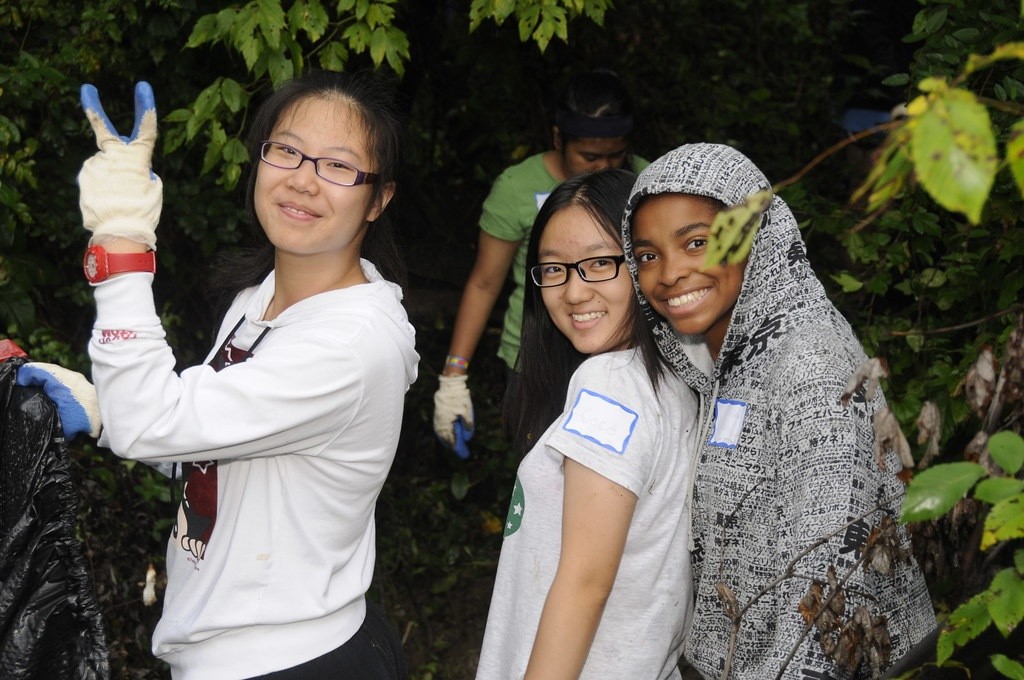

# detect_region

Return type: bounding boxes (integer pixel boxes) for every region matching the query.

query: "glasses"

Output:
[532,255,625,288]
[258,141,379,187]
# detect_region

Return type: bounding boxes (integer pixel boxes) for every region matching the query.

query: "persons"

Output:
[431,88,652,457]
[15,70,420,680]
[620,142,937,680]
[472,165,700,680]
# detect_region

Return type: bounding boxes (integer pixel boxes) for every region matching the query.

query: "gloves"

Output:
[75,81,163,252]
[432,375,475,458]
[15,362,103,442]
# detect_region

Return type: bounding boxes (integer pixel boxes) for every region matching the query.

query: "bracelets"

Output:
[445,354,469,369]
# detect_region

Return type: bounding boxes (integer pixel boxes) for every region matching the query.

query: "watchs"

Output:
[84,243,157,283]
